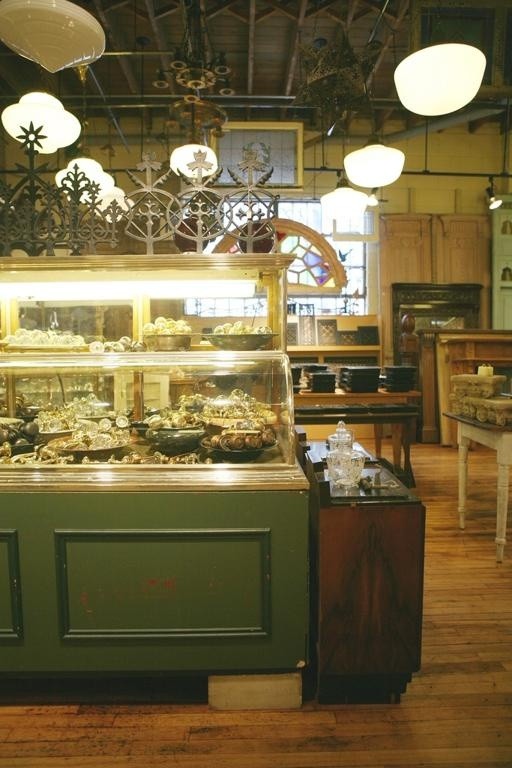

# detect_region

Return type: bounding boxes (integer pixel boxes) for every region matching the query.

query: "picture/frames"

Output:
[209,120,305,193]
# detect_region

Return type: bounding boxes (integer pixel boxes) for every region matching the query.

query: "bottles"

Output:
[327,420,355,451]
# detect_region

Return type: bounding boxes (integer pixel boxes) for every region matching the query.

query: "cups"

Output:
[325,448,366,488]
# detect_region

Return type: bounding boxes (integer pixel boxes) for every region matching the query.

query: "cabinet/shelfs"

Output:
[376,210,491,438]
[1,253,426,711]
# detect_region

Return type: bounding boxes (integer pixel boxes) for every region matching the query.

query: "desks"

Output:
[442,409,512,563]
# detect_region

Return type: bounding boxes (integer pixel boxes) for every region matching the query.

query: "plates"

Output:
[46,435,137,460]
[198,434,280,461]
[289,364,418,393]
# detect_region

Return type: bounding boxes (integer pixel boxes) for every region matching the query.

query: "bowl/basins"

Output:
[199,332,280,351]
[33,428,79,443]
[146,426,209,457]
[139,332,196,352]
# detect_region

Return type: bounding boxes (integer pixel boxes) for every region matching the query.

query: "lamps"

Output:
[54,158,114,199]
[1,89,82,157]
[168,141,218,181]
[319,2,487,221]
[1,0,106,74]
[90,186,135,223]
